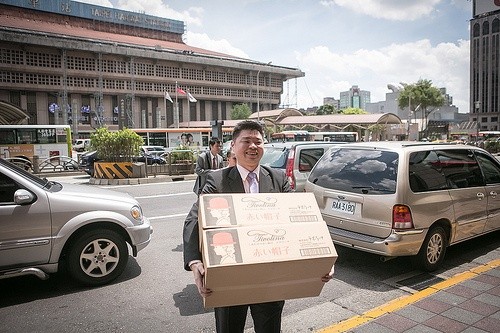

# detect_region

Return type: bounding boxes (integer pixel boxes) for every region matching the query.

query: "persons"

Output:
[181,133,194,146]
[192,137,237,196]
[182,121,335,333]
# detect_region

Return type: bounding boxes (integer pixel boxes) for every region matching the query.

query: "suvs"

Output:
[0,158,153,288]
[140,144,165,155]
[260,141,351,193]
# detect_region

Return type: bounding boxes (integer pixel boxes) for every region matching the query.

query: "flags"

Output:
[165,92,173,103]
[177,86,186,95]
[188,93,197,102]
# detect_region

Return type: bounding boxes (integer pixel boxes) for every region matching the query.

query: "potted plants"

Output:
[169,144,196,174]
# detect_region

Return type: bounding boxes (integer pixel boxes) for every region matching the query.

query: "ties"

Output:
[213,157,217,170]
[246,172,260,193]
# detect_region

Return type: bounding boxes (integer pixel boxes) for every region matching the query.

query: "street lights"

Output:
[472,101,481,140]
[256,61,272,122]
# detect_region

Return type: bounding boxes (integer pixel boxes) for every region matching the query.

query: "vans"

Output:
[73,138,91,152]
[304,139,498,267]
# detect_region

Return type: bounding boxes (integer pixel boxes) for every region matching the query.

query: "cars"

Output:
[80,149,167,178]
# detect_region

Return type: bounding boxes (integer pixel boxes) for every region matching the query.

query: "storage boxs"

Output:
[198,193,338,309]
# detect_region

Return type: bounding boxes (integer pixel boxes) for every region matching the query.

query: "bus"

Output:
[0,125,73,172]
[272,129,359,143]
